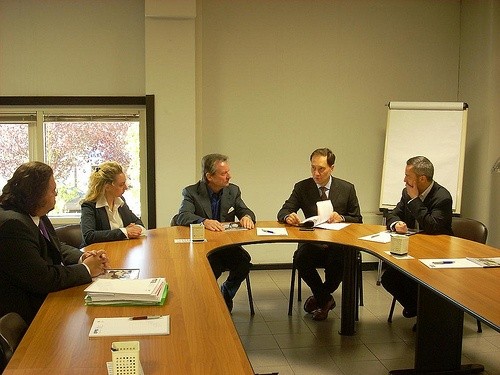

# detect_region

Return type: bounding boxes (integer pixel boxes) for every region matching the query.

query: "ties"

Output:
[39,220,50,241]
[320,186,328,201]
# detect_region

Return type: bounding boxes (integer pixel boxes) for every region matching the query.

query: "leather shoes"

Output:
[312,295,336,321]
[304,295,319,312]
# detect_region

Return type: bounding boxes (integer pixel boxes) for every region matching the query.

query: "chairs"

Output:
[170,214,256,317]
[288,244,364,321]
[386,218,487,333]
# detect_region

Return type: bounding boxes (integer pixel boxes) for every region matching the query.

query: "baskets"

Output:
[111,341,139,375]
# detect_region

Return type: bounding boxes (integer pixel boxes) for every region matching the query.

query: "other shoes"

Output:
[402,309,416,317]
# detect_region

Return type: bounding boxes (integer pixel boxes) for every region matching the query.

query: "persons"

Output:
[385,155,453,332]
[175,154,256,314]
[0,160,111,328]
[80,161,145,247]
[277,148,363,320]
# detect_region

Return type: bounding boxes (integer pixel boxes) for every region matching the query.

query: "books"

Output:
[297,215,351,230]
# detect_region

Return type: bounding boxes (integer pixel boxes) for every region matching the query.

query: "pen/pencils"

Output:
[300,229,314,231]
[390,235,394,237]
[432,261,454,264]
[128,315,161,320]
[371,235,379,238]
[262,229,273,233]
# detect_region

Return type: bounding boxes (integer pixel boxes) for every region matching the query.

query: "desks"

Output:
[0,221,500,375]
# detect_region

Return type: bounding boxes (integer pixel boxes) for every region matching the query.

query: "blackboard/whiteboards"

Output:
[376,101,469,217]
